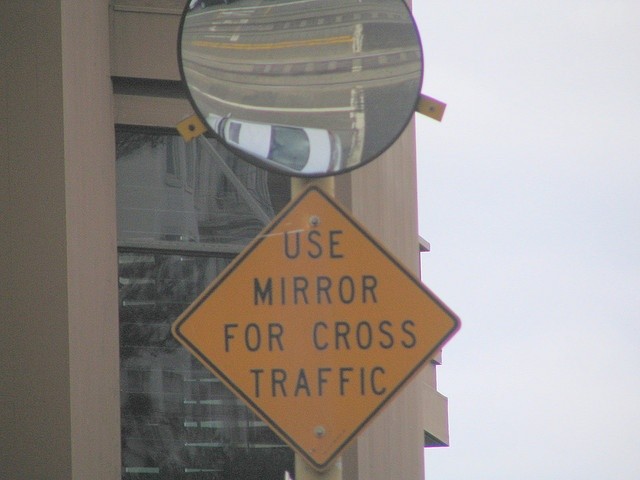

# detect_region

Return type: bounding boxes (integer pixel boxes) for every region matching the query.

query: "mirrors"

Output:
[177,0,423,177]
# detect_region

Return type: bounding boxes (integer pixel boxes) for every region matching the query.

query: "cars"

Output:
[207,114,342,177]
